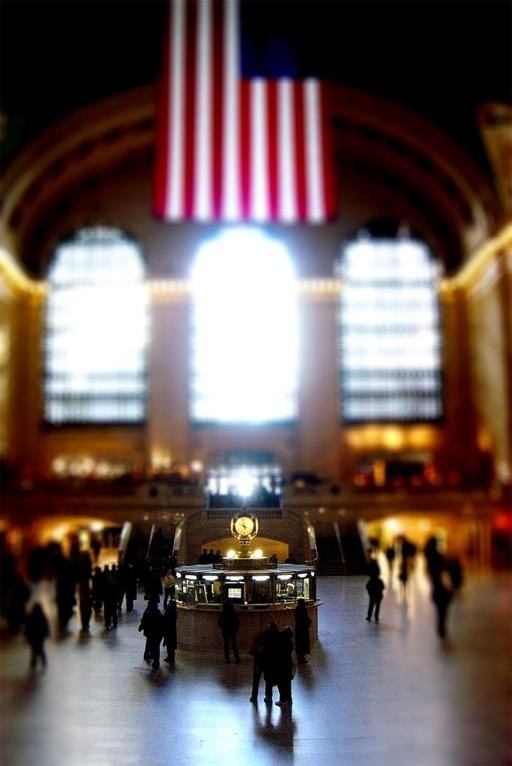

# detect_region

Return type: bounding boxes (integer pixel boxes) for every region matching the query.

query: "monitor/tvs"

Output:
[224,581,247,604]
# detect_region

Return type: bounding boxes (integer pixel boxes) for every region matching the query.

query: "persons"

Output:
[0,534,180,672]
[293,599,312,667]
[364,531,463,642]
[249,623,294,706]
[270,554,278,563]
[199,549,222,565]
[284,554,298,564]
[219,601,242,663]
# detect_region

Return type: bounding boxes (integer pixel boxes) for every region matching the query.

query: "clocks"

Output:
[231,511,259,543]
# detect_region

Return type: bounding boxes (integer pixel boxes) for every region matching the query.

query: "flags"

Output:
[152,0,337,224]
